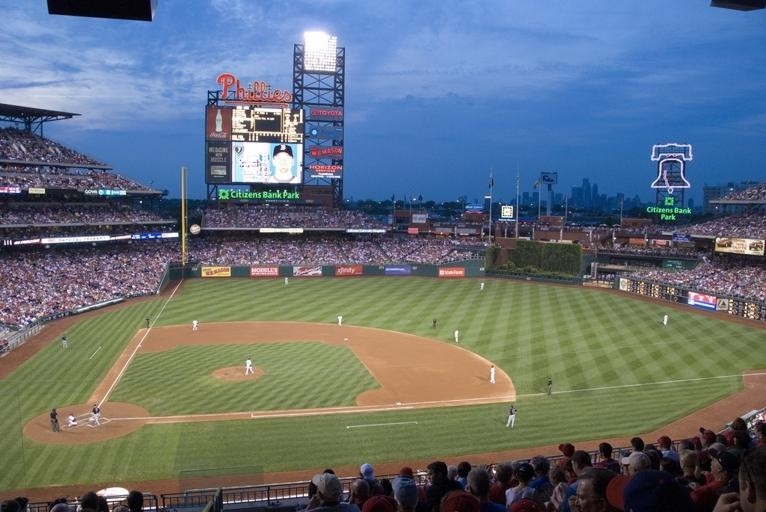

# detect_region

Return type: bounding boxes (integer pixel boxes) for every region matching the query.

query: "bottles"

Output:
[215,109,223,132]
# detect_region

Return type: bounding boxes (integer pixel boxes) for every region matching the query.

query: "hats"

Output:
[400,467,413,477]
[360,463,376,481]
[362,495,398,511]
[311,473,343,499]
[606,469,679,512]
[702,443,726,456]
[699,426,716,440]
[622,451,651,470]
[443,492,479,512]
[558,443,575,454]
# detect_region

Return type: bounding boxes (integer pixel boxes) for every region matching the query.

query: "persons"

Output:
[490,364,496,384]
[66,412,78,428]
[304,416,766,511]
[179,180,766,321]
[91,404,102,426]
[1,124,181,358]
[50,408,61,432]
[244,356,255,376]
[0,488,144,511]
[454,328,460,343]
[432,318,437,330]
[505,403,519,429]
[546,377,553,399]
[266,143,301,185]
[661,313,669,327]
[191,318,199,333]
[337,313,343,328]
